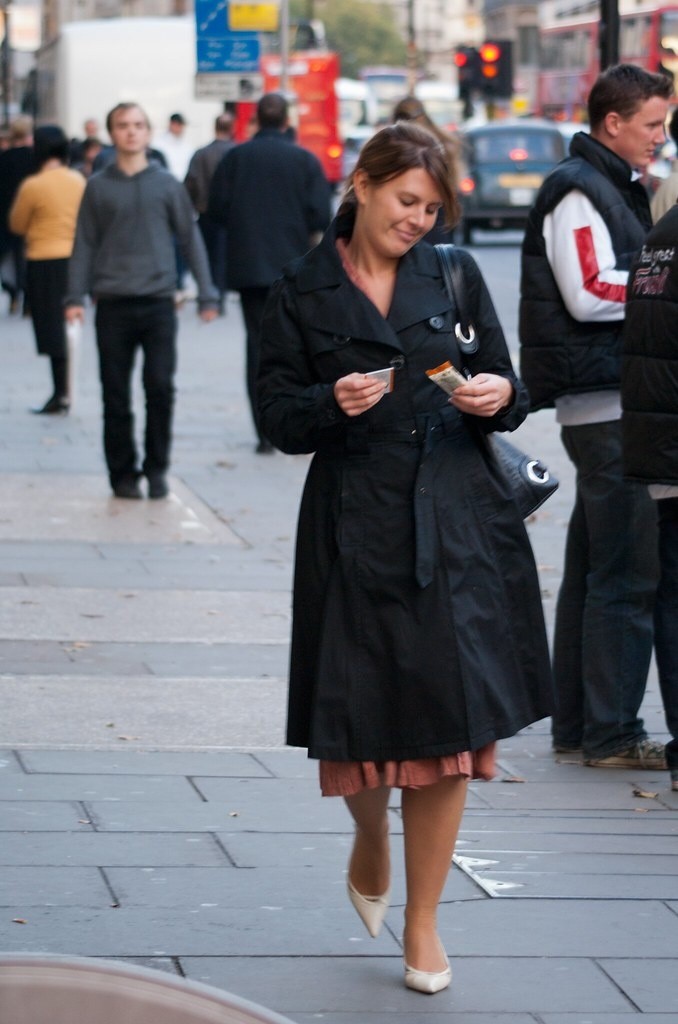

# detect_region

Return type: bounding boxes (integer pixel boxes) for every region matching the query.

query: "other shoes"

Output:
[256,438,274,453]
[147,470,169,499]
[669,767,678,791]
[11,284,22,314]
[34,396,72,415]
[112,478,142,500]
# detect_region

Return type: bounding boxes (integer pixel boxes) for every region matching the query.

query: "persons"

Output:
[198,94,332,456]
[184,112,238,214]
[393,97,461,241]
[258,122,559,993]
[520,64,666,769]
[282,126,296,142]
[623,108,678,790]
[66,137,102,177]
[0,112,34,319]
[149,114,201,183]
[8,125,87,416]
[93,147,188,308]
[61,100,219,499]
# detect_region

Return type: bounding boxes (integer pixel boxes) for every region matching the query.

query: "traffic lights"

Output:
[455,47,478,99]
[478,40,511,97]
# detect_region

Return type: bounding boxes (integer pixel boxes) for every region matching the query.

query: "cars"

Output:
[454,124,568,245]
[334,66,485,172]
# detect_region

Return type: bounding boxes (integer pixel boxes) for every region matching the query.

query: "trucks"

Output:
[19,18,224,176]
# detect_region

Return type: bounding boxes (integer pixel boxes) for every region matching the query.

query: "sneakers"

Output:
[579,736,671,769]
[551,737,585,753]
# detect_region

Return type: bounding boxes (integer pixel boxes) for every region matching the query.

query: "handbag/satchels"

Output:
[431,240,561,519]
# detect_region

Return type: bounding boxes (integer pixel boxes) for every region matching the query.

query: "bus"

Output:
[534,1,678,127]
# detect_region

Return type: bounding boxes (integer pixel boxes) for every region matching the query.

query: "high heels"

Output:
[346,867,391,939]
[402,923,454,993]
